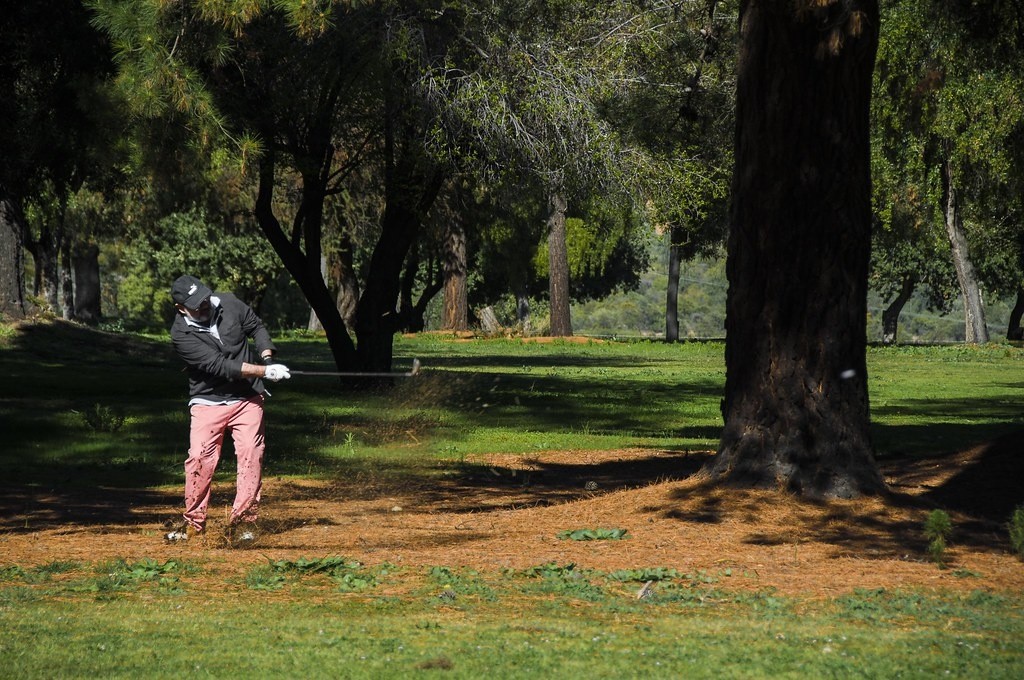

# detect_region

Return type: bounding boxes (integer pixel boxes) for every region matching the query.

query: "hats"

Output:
[171,275,212,310]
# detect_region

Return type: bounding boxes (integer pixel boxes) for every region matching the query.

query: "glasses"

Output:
[193,296,209,312]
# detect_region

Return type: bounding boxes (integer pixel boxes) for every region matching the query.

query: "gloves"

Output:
[264,364,291,383]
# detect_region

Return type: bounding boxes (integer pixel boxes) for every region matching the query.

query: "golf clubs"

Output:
[285,356,420,378]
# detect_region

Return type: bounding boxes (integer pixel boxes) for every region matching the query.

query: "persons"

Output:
[167,275,294,544]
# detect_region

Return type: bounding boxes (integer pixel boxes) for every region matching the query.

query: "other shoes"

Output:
[167,523,205,540]
[225,524,254,543]
